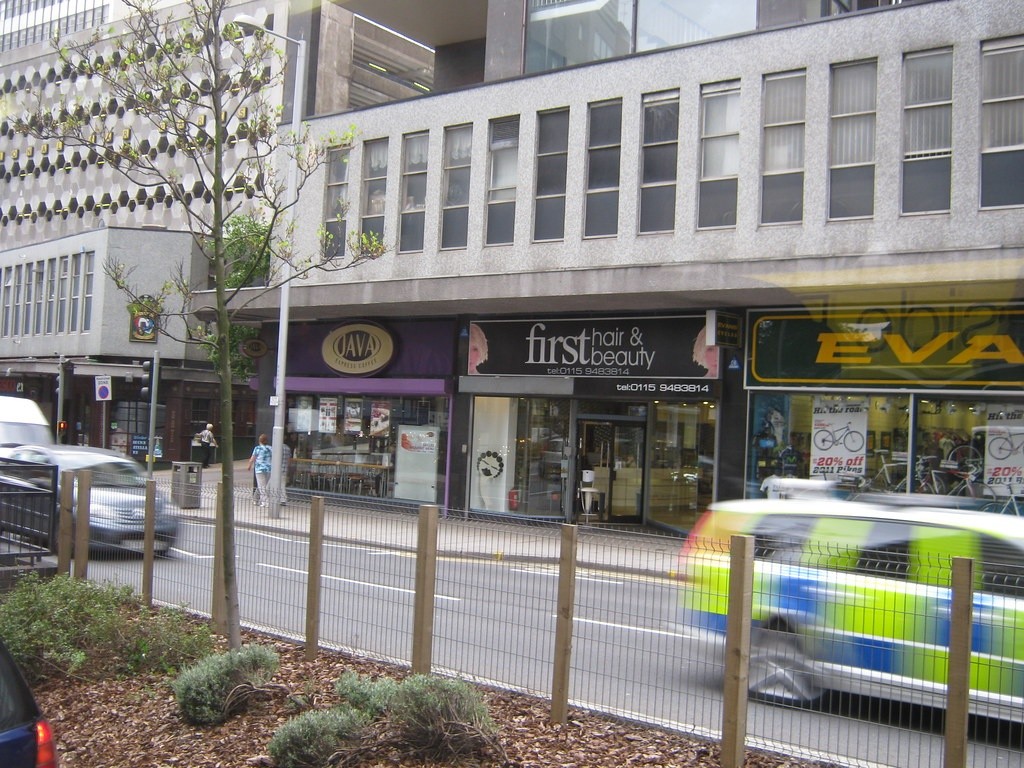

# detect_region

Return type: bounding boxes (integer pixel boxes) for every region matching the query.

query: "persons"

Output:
[248,434,272,506]
[199,423,218,468]
[266,435,291,506]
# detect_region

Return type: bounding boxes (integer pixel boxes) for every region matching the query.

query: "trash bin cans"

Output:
[171,461,203,508]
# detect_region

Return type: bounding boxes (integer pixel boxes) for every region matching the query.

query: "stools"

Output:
[291,471,374,495]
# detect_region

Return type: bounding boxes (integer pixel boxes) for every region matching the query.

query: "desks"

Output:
[285,459,390,496]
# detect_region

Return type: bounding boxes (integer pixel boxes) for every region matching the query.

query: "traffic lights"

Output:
[141,361,151,398]
[58,421,67,437]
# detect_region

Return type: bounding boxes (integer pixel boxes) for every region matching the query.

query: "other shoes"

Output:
[252,500,257,505]
[280,502,286,506]
[260,503,269,508]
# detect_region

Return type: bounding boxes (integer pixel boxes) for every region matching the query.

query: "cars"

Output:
[0,445,180,559]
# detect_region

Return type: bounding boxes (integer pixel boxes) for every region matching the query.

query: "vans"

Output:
[0,396,54,459]
[673,499,1024,724]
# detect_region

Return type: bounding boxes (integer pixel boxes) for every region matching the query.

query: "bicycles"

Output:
[847,433,1024,516]
